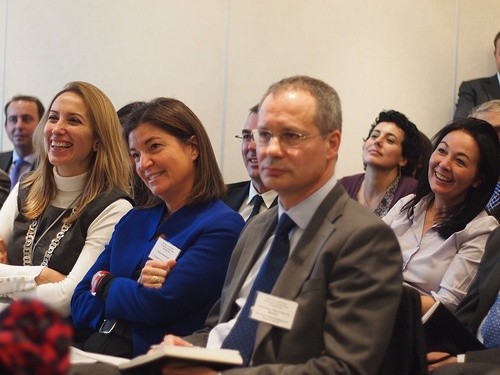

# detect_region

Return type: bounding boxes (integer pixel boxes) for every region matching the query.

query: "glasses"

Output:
[250,128,340,148]
[235,133,251,143]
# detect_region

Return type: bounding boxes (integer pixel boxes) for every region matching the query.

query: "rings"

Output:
[150,274,161,282]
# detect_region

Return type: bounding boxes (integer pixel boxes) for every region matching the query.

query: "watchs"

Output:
[89,271,112,296]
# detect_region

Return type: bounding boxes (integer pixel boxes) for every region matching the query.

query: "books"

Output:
[68,344,244,369]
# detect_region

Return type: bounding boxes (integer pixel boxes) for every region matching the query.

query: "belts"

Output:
[99,317,135,341]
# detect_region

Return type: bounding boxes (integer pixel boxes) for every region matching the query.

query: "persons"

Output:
[0,76,500,375]
[336,109,421,219]
[453,32,500,121]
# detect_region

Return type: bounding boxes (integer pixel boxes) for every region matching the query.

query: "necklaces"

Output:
[21,193,81,267]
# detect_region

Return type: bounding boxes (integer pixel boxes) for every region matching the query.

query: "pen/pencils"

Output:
[432,349,465,364]
[151,344,202,349]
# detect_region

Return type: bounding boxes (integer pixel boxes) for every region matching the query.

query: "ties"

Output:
[245,195,264,223]
[221,212,298,369]
[481,294,500,347]
[9,160,28,192]
